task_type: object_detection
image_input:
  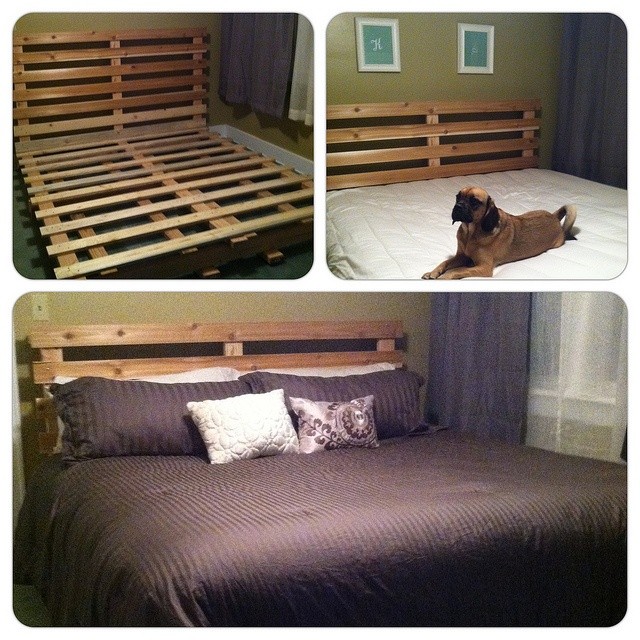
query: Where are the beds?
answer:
[328,101,628,280]
[14,31,315,282]
[30,325,628,627]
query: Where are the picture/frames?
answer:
[354,16,402,74]
[457,22,494,76]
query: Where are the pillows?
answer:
[187,387,300,464]
[51,376,254,462]
[288,395,379,453]
[239,367,424,438]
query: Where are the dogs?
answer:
[421,187,576,280]
